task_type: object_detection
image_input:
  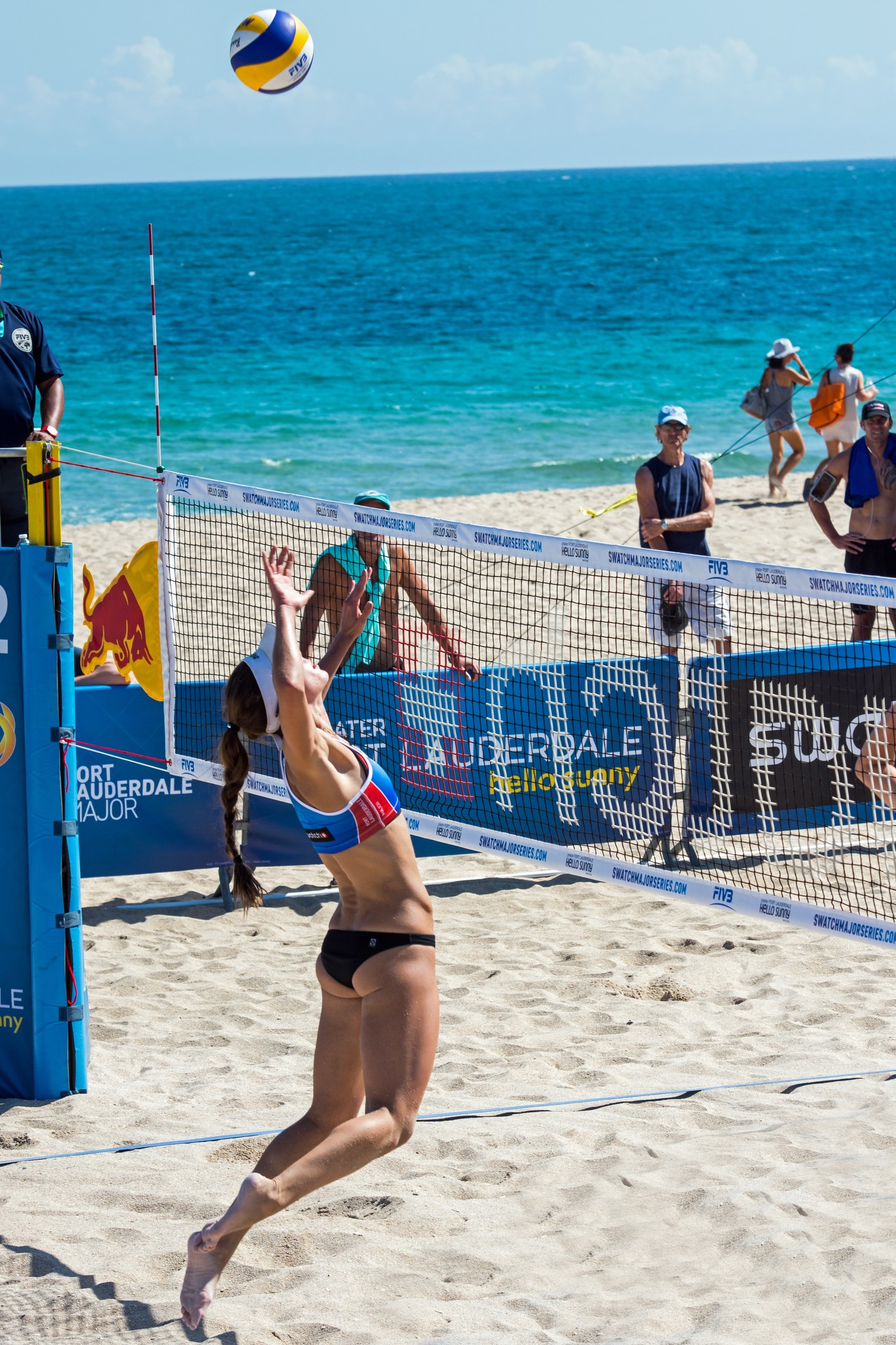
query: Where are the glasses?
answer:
[660,422,686,431]
[658,405,688,425]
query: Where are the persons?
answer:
[759,337,812,500]
[70,645,130,686]
[179,545,440,1330]
[0,248,66,549]
[802,343,878,503]
[300,490,483,683]
[807,401,896,643]
[636,405,732,656]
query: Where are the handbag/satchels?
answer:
[739,368,769,421]
[808,369,846,430]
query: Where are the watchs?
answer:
[661,518,668,532]
[40,427,58,440]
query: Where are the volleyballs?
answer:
[231,8,314,94]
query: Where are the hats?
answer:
[354,490,392,511]
[862,401,890,421]
[239,621,284,752]
[766,339,800,359]
